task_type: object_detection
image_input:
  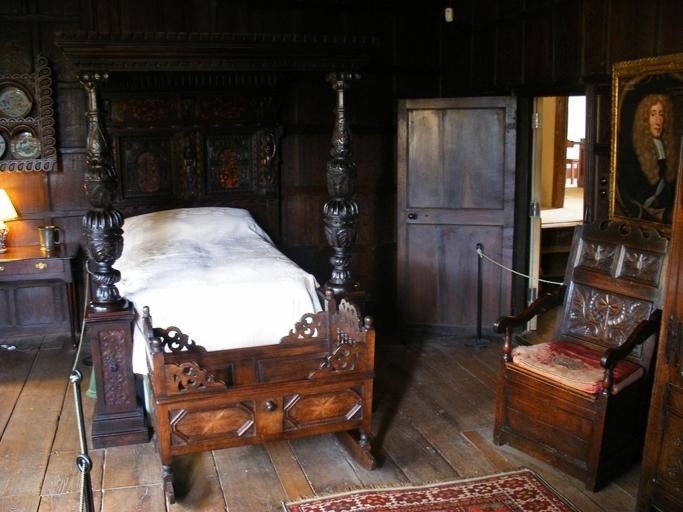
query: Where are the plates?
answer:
[0,125,41,161]
[0,82,34,121]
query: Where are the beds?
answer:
[113,208,378,504]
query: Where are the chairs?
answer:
[493,221,670,493]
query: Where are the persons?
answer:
[614,92,674,222]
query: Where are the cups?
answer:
[39,225,61,250]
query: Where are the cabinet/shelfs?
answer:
[526,225,575,332]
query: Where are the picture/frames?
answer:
[607,52,682,239]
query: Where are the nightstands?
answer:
[0,243,81,353]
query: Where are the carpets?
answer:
[279,465,584,512]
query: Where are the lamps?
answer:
[0,189,19,254]
